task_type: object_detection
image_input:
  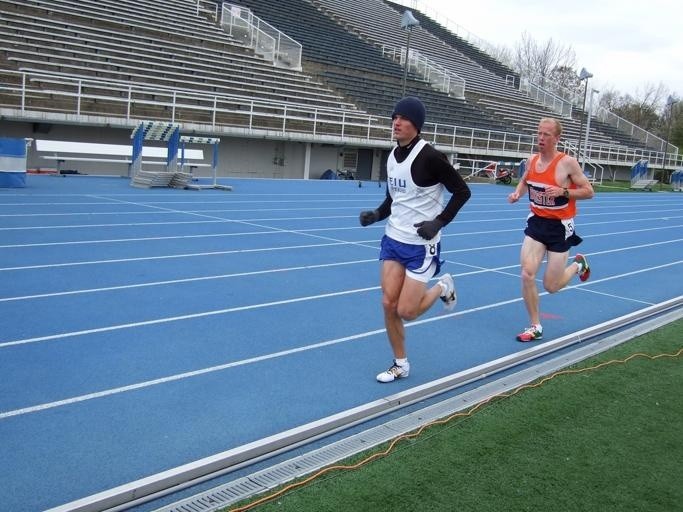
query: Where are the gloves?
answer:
[360,210,375,227]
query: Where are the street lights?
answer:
[578,65,593,156]
[662,94,677,185]
[400,9,419,99]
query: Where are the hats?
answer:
[391,96,427,135]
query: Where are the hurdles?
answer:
[131,119,233,191]
[495,158,530,178]
[629,159,657,192]
[670,169,683,192]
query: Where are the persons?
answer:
[508,117,596,342]
[360,97,473,384]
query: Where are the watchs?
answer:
[562,187,571,200]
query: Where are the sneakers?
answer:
[375,359,410,383]
[573,253,590,282]
[439,273,457,311]
[515,325,544,342]
[413,220,440,241]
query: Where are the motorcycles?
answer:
[485,163,512,184]
